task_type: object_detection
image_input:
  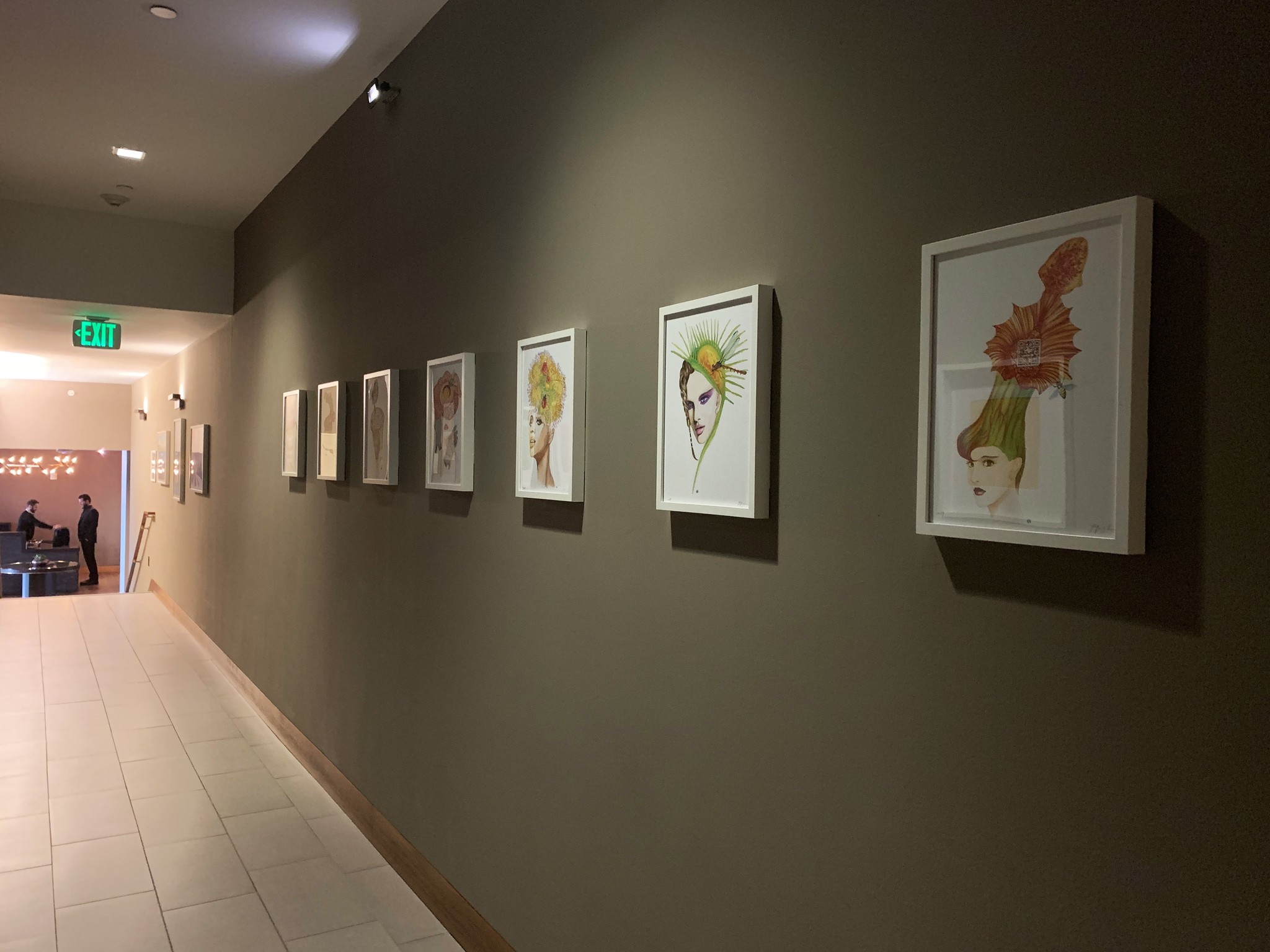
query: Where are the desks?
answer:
[0,558,81,597]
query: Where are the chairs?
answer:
[37,527,69,548]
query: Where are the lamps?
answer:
[0,452,80,478]
[168,394,184,409]
[135,409,146,420]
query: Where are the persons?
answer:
[16,499,62,542]
[78,494,99,586]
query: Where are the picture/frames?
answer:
[156,430,170,485]
[150,451,157,482]
[172,419,186,502]
[358,368,401,487]
[313,380,347,482]
[420,349,480,493]
[509,324,591,509]
[188,421,211,495]
[653,280,778,523]
[911,189,1163,562]
[279,389,307,479]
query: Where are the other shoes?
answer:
[80,578,96,585]
